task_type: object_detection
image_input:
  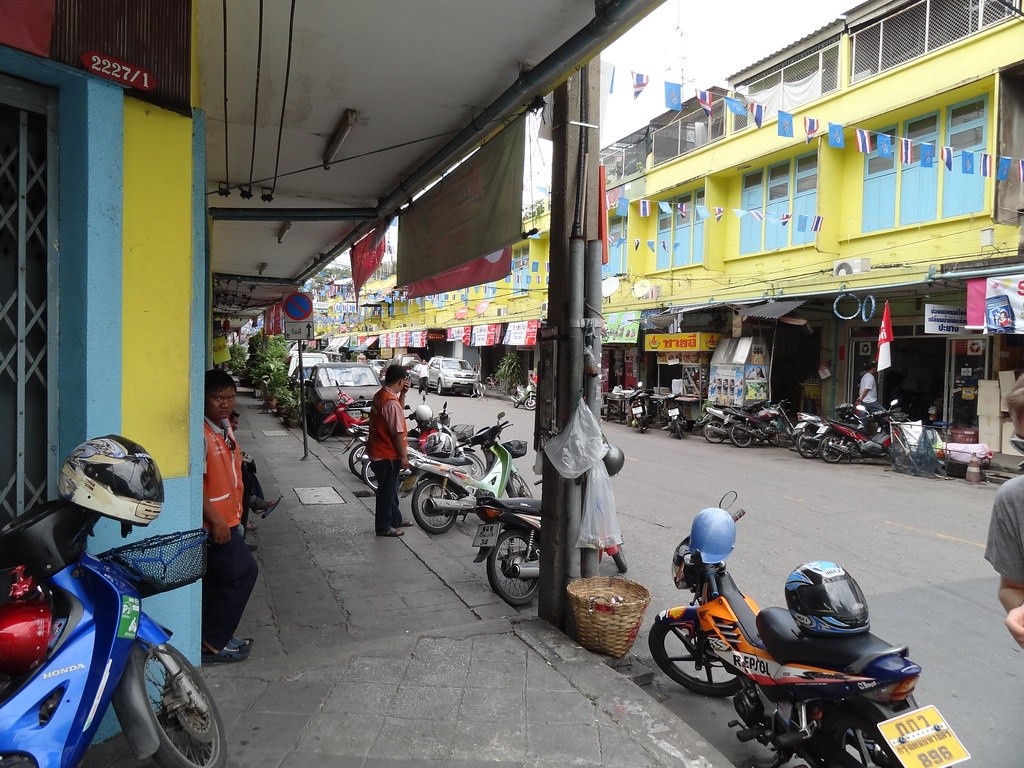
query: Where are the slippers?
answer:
[262,495,282,518]
[202,638,253,662]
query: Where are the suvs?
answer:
[305,361,383,436]
[427,355,480,397]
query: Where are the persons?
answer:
[709,367,766,399]
[984,374,1024,653]
[996,311,1011,326]
[202,369,259,664]
[417,359,431,395]
[856,364,888,435]
[366,365,414,537]
[228,411,282,551]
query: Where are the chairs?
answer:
[356,373,371,384]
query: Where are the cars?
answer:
[284,350,429,410]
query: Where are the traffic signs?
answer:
[284,321,314,341]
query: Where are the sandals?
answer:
[400,519,414,527]
[377,530,405,537]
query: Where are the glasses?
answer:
[1009,432,1024,454]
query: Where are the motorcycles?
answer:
[646,489,972,768]
[510,377,536,411]
[627,381,654,434]
[314,380,369,442]
[697,398,912,464]
[472,477,629,609]
[657,391,688,440]
[341,394,476,496]
[405,411,534,535]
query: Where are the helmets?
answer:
[59,435,164,526]
[689,508,736,563]
[0,600,51,672]
[786,562,869,636]
[427,432,452,457]
[416,405,432,420]
[603,444,624,475]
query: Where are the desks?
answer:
[602,390,698,425]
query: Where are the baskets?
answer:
[98,528,207,597]
[501,440,527,458]
[567,574,651,657]
[450,424,474,442]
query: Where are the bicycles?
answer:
[483,373,506,392]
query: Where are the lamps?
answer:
[259,262,268,275]
[323,108,357,171]
[277,222,291,244]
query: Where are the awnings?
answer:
[287,335,379,352]
[628,303,727,322]
[739,300,808,319]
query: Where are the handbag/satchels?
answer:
[576,461,623,548]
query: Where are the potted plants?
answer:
[495,352,524,396]
[227,337,306,427]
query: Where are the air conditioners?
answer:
[400,324,406,328]
[496,307,507,316]
[412,323,416,327]
[541,302,548,312]
[833,257,871,277]
[642,286,663,300]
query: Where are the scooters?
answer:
[1,499,232,768]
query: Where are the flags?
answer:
[876,302,894,372]
[599,60,1023,182]
[605,195,823,251]
[300,244,549,325]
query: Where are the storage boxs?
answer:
[977,369,1024,457]
